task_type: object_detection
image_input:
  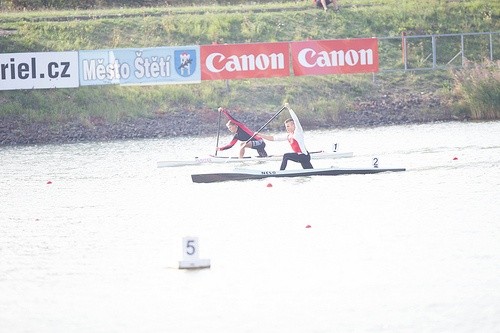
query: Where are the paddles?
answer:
[211,108,221,158]
[239,106,285,158]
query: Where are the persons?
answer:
[255,103,315,170]
[216,107,274,158]
[313,0,341,13]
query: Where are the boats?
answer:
[190,157,407,183]
[154,142,355,167]
